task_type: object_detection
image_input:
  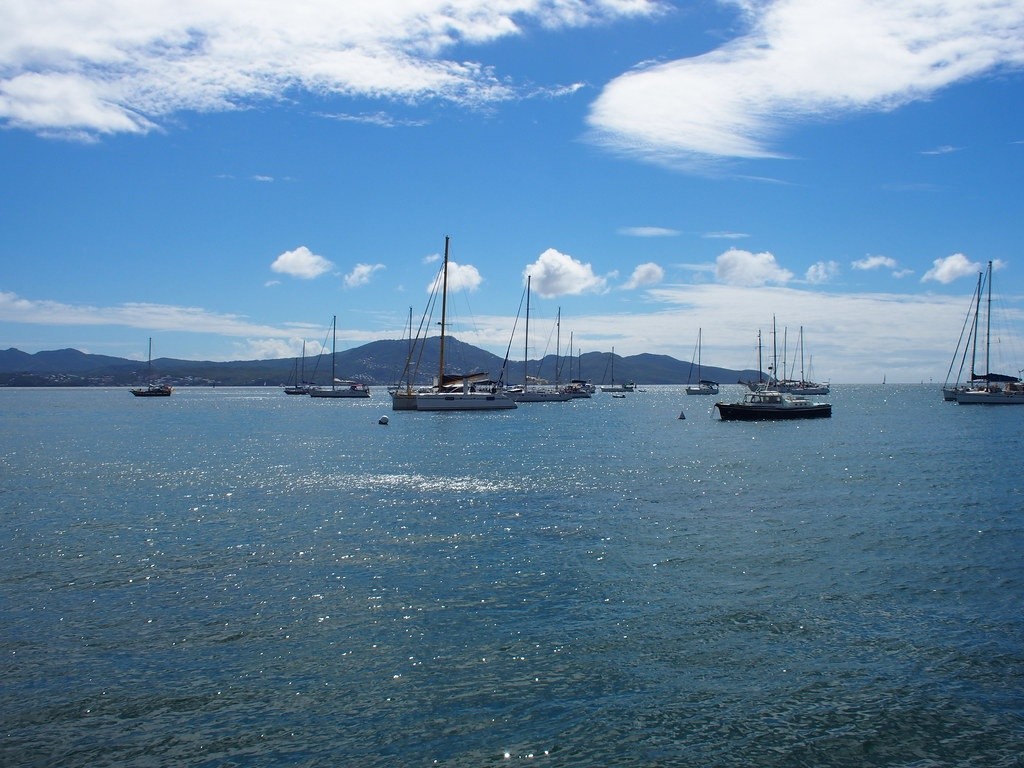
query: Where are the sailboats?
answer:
[388,235,454,410]
[128,337,172,396]
[307,316,373,398]
[599,346,634,392]
[684,328,719,396]
[492,275,572,402]
[285,358,303,389]
[956,261,1024,404]
[283,340,308,394]
[528,307,591,398]
[941,272,984,402]
[748,312,801,392]
[882,373,886,384]
[389,308,419,397]
[787,326,830,395]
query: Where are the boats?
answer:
[714,391,832,422]
[622,380,637,388]
[612,394,626,398]
[416,378,518,410]
[563,379,596,393]
[638,389,648,392]
